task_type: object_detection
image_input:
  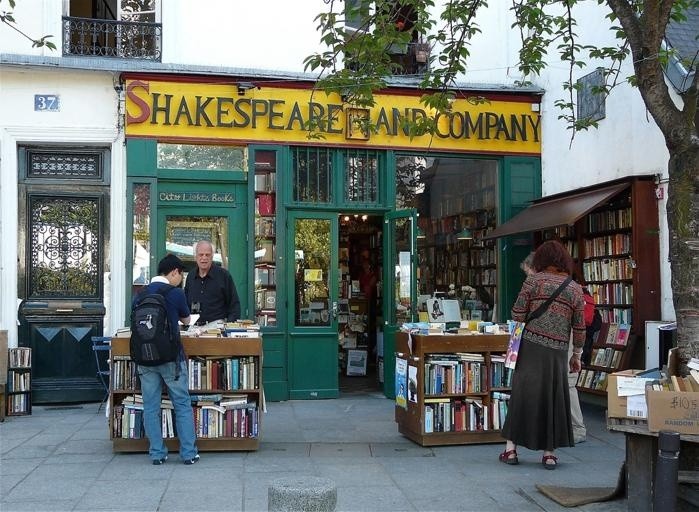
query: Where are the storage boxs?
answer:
[609,369,699,434]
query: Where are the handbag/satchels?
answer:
[505,321,525,370]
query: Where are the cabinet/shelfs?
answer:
[4,347,33,415]
[110,337,262,454]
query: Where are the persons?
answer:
[497,240,588,470]
[183,240,242,331]
[128,253,201,467]
[517,249,602,446]
[359,258,377,297]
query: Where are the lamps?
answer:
[237,80,254,93]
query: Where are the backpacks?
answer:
[129,284,180,366]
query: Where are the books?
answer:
[403,321,520,336]
[416,168,496,320]
[424,352,514,433]
[637,346,698,397]
[540,195,637,392]
[8,346,32,414]
[340,231,360,298]
[255,148,331,327]
[107,311,263,441]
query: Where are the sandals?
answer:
[541,455,557,469]
[499,449,518,464]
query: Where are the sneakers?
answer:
[151,456,168,465]
[184,454,200,464]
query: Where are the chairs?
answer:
[91,335,112,414]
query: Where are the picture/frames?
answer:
[165,222,217,261]
